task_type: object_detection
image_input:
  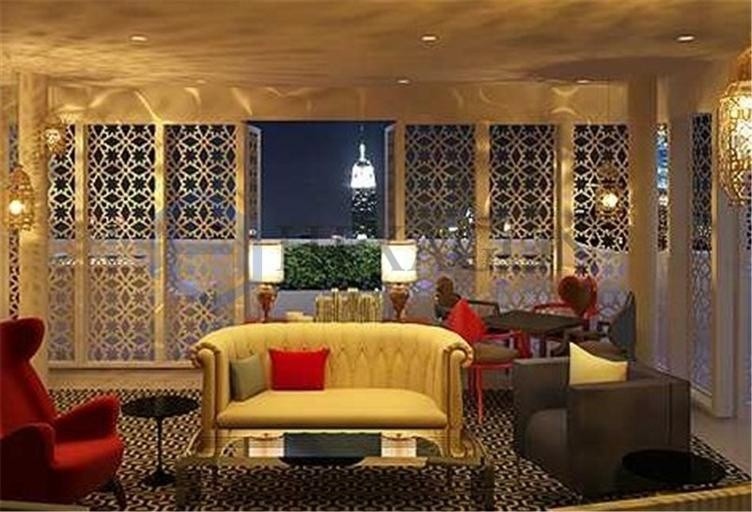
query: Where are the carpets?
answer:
[45,389,750,512]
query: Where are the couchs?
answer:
[191,322,474,459]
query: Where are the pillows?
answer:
[229,354,267,400]
[269,348,330,390]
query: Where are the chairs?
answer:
[435,275,636,423]
[513,354,689,497]
[0,319,124,511]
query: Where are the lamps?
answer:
[595,75,621,219]
[381,241,419,321]
[247,240,284,321]
[39,75,66,157]
[718,50,751,208]
[0,73,34,233]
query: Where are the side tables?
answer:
[122,396,199,487]
[619,449,726,493]
